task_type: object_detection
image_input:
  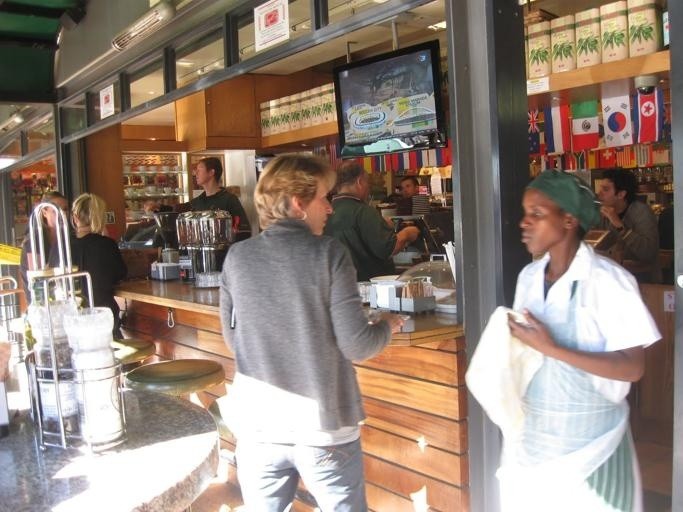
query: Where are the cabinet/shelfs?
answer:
[123,171,185,225]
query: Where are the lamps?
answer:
[14,113,24,124]
[111,0,177,53]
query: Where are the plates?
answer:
[369,274,413,282]
[411,192,431,217]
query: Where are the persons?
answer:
[19,191,71,313]
[592,167,661,284]
[55,193,124,342]
[217,153,405,511]
[323,158,419,281]
[399,177,419,214]
[497,170,647,512]
[143,154,250,268]
[657,202,675,251]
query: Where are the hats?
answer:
[526,170,600,232]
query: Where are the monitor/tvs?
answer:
[333,38,447,159]
[390,215,442,254]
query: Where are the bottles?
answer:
[0,288,122,446]
[661,1,670,51]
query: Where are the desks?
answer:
[0,388,220,512]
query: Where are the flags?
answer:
[526,87,662,155]
[313,144,451,170]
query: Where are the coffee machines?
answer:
[152,212,185,262]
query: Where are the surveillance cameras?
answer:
[634,74,660,95]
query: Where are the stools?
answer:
[124,359,226,400]
[110,338,156,366]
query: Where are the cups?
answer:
[123,163,181,218]
